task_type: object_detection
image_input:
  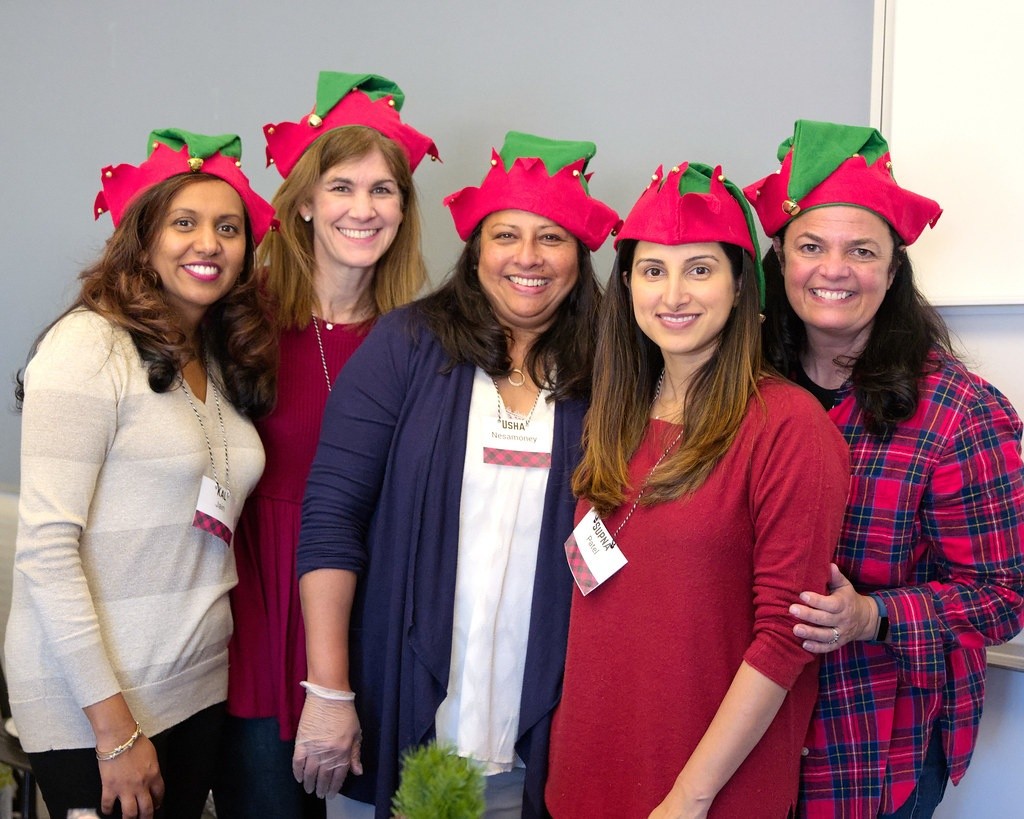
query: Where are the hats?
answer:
[613,161,766,323]
[741,118,943,247]
[263,71,443,183]
[94,128,282,252]
[442,129,619,252]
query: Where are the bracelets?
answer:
[95,720,142,761]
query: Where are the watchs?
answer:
[864,592,890,646]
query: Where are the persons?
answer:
[4,127,266,819]
[742,119,1024,819]
[210,71,444,819]
[293,130,625,819]
[544,161,851,819]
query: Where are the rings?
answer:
[828,628,839,645]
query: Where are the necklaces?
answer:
[312,295,380,330]
[507,369,526,387]
[655,370,686,419]
